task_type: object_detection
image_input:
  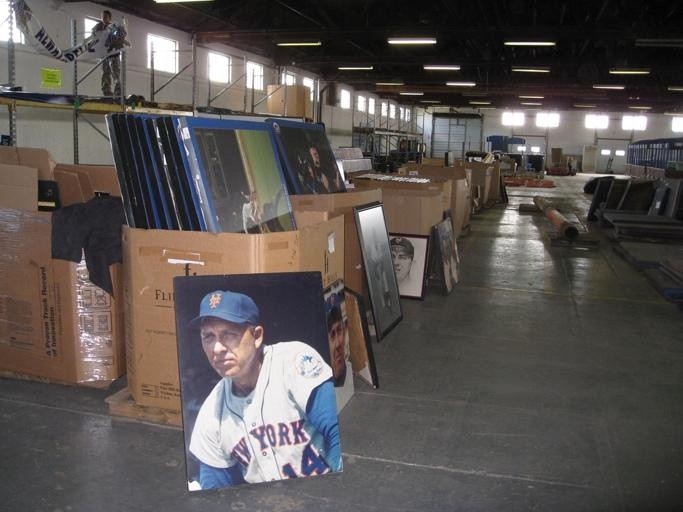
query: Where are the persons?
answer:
[325,306,355,415]
[187,289,343,490]
[389,237,418,295]
[309,145,328,176]
[440,235,458,294]
[91,10,121,96]
[329,286,339,306]
[370,225,394,314]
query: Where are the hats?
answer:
[191,290,262,328]
[390,236,415,253]
[325,294,342,314]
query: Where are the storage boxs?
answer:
[263,81,309,118]
